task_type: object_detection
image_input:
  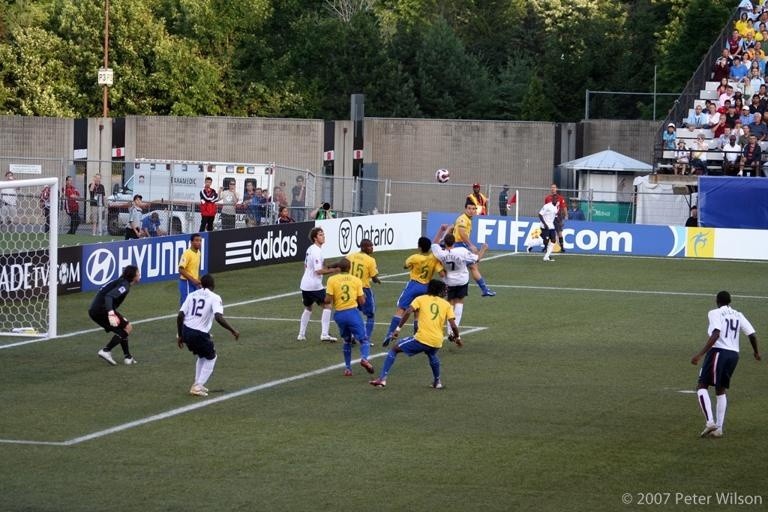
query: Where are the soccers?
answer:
[436,169,449,183]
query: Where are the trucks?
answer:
[97,157,275,237]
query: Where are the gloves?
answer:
[108,311,120,327]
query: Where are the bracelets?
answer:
[107,313,115,316]
[456,336,460,340]
[395,326,400,331]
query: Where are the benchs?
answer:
[658,48,767,176]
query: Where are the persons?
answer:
[367,277,464,390]
[88,264,143,365]
[346,238,383,347]
[39,184,61,232]
[525,194,561,263]
[542,184,569,253]
[323,258,375,376]
[88,173,107,237]
[124,193,144,240]
[383,235,447,348]
[140,212,162,238]
[685,205,698,228]
[175,275,240,397]
[430,223,488,347]
[179,234,201,309]
[296,227,337,342]
[0,171,22,226]
[662,0,768,177]
[567,198,585,221]
[691,291,761,438]
[61,176,82,235]
[464,181,488,216]
[497,184,510,215]
[198,172,337,240]
[450,201,497,297]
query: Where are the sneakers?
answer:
[430,384,442,389]
[98,349,116,366]
[344,369,352,376]
[481,291,496,296]
[527,245,532,253]
[383,335,392,347]
[321,335,337,343]
[192,385,208,397]
[448,334,455,342]
[543,257,555,262]
[361,360,374,373]
[297,335,306,341]
[124,355,137,365]
[369,380,386,387]
[711,431,723,438]
[701,425,718,438]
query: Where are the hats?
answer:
[677,140,686,145]
[473,184,480,187]
[742,106,749,110]
[668,123,675,128]
